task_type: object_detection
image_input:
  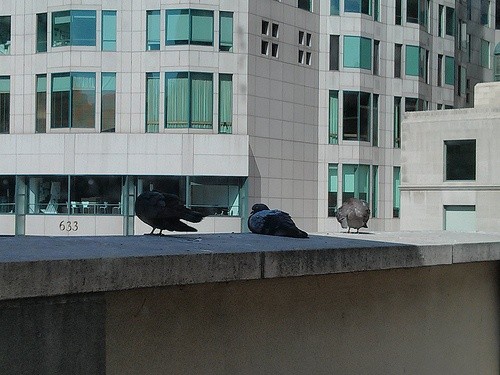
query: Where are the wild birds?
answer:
[134,190,208,237]
[247,204,308,238]
[335,197,370,235]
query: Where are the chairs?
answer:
[82,201,90,214]
[100,201,108,214]
[71,201,79,214]
[111,202,120,214]
